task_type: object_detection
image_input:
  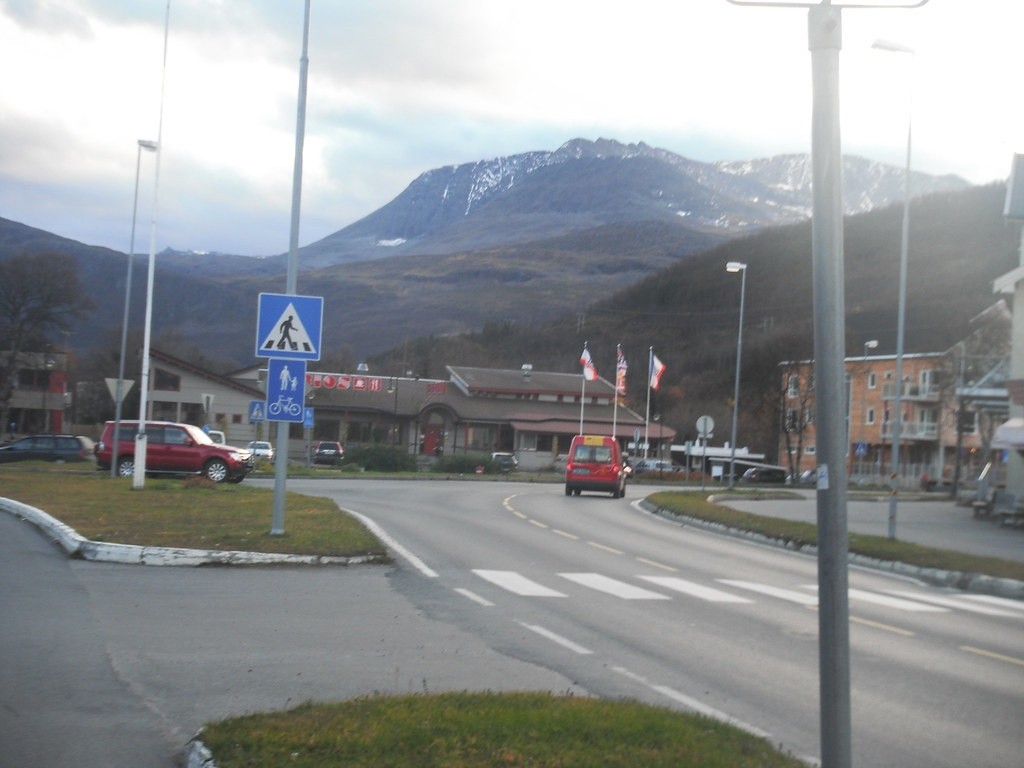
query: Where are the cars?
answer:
[0,435,95,461]
[627,457,687,473]
[246,441,276,463]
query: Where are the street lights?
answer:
[453,422,460,457]
[872,38,917,540]
[387,374,398,452]
[111,140,158,480]
[726,262,747,489]
[413,419,422,455]
[858,341,879,483]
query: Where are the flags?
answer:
[580,347,597,381]
[649,353,666,391]
[616,349,627,408]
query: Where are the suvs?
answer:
[490,452,518,472]
[314,441,344,464]
[97,421,254,483]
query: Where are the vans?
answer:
[565,435,627,498]
[205,431,225,445]
[742,468,786,484]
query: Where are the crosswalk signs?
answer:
[249,401,265,423]
[255,293,323,361]
